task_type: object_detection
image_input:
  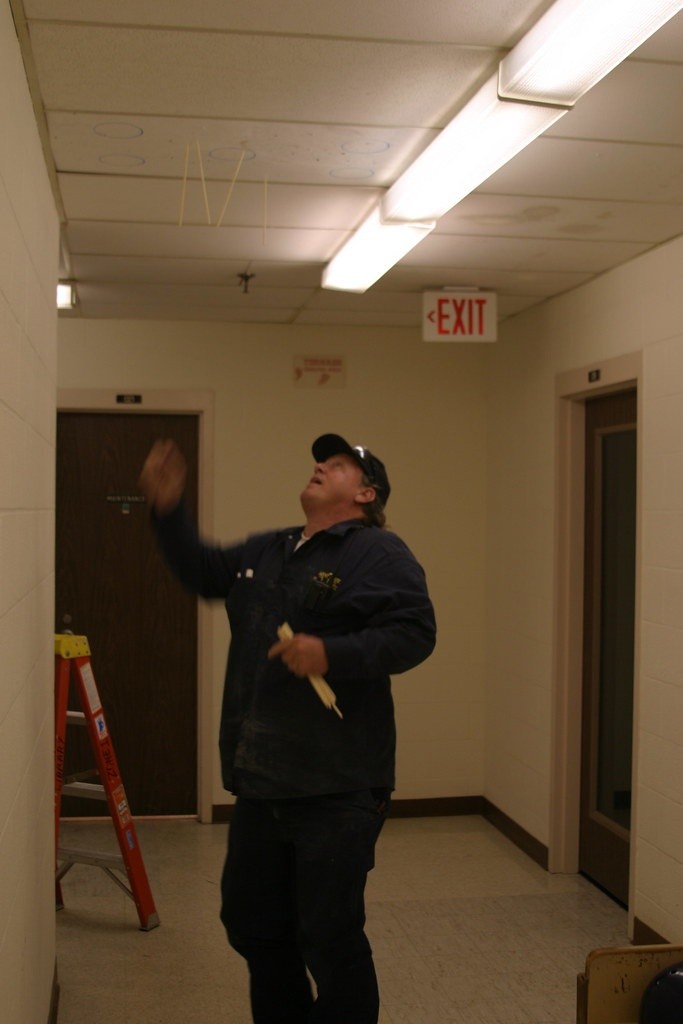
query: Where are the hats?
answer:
[312,433,391,504]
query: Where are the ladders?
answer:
[54,634,161,933]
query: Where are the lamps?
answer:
[317,0,682,294]
[56,279,78,310]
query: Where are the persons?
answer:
[141,433,438,1024]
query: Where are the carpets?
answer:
[362,890,634,1024]
